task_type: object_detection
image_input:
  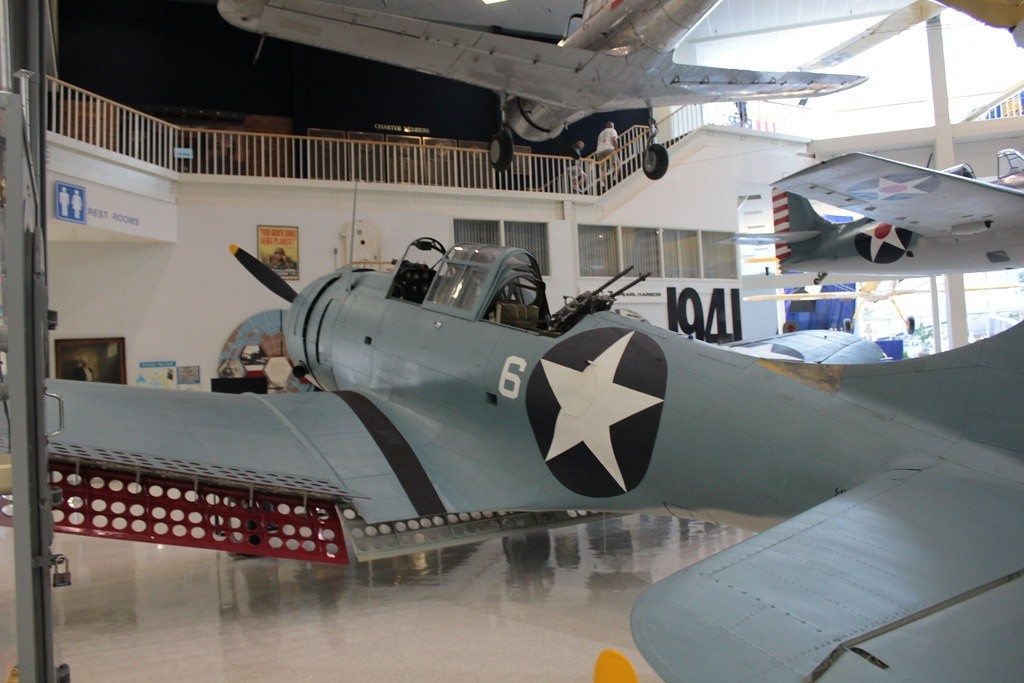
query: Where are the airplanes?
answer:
[720,146,1024,277]
[48,238,1024,683]
[216,0,871,180]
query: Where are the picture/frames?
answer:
[257,225,299,281]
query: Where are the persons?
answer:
[567,141,587,195]
[596,121,620,193]
